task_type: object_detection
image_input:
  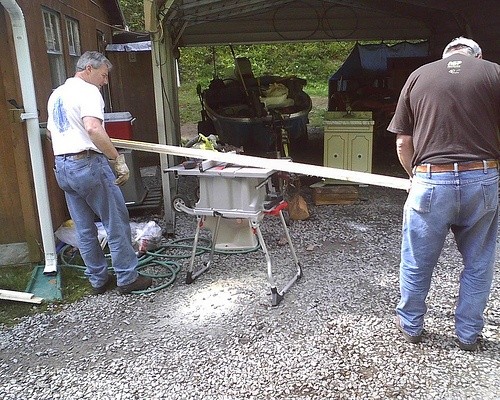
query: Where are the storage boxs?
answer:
[105,111,136,141]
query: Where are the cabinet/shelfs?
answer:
[320,109,375,187]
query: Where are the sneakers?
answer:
[121,273,151,293]
[92,274,115,294]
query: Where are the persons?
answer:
[387,35,499,351]
[47,50,153,296]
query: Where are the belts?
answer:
[59,150,99,156]
[415,160,497,173]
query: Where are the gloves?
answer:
[108,154,129,186]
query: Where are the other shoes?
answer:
[396,316,421,343]
[460,341,477,351]
